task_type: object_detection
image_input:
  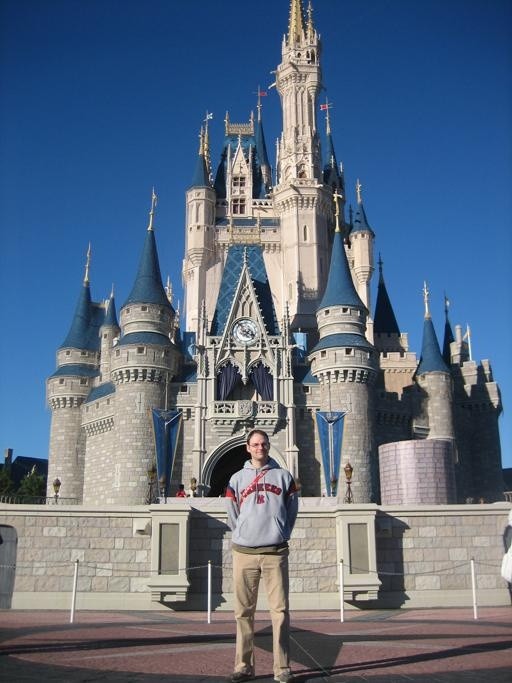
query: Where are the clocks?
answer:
[232,318,258,344]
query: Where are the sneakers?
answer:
[273,672,295,683]
[230,671,255,682]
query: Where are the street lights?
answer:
[148,465,155,504]
[344,462,355,506]
[53,478,63,502]
[159,472,170,504]
[191,477,197,495]
[329,474,337,497]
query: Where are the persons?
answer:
[225,431,298,683]
[185,478,198,497]
[176,484,186,498]
